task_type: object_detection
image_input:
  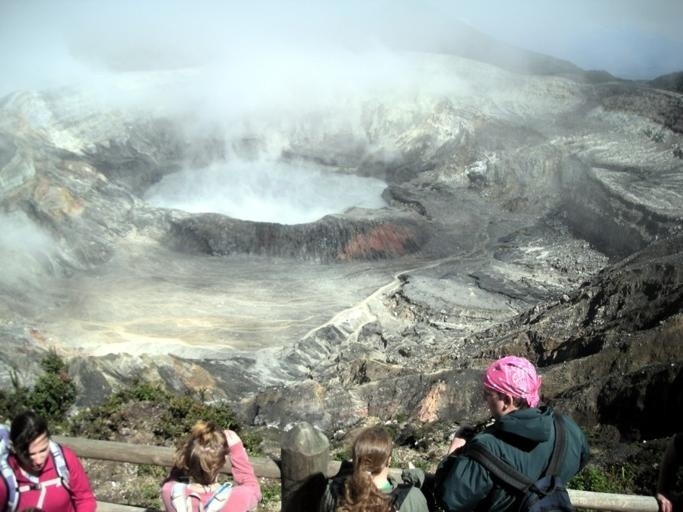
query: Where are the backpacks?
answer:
[459,411,573,511]
[1,426,70,512]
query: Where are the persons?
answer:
[431,355,590,512]
[0,411,98,512]
[318,426,431,512]
[160,419,262,512]
[654,430,683,512]
